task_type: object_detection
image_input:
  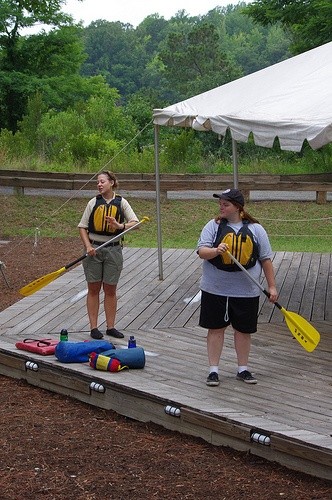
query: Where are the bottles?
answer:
[128,335,136,349]
[60,329,68,342]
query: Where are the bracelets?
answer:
[123,223,125,229]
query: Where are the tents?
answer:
[153,41,332,282]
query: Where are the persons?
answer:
[197,188,279,386]
[77,170,140,339]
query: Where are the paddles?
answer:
[224,248,321,352]
[19,216,150,297]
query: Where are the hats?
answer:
[213,189,245,206]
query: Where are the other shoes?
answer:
[91,328,103,339]
[206,372,220,385]
[236,370,258,384]
[106,328,124,338]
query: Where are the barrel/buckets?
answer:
[316,190,327,204]
